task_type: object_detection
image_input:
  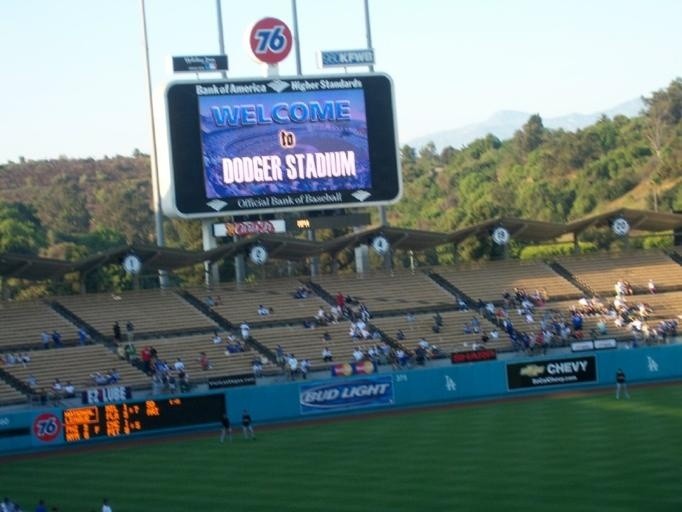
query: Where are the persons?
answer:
[349,317,409,364]
[416,338,444,364]
[36,499,48,512]
[91,368,118,386]
[99,496,112,512]
[504,286,608,351]
[456,296,512,350]
[303,292,370,329]
[298,285,308,298]
[607,291,675,346]
[240,408,257,441]
[6,351,38,384]
[649,279,657,294]
[42,327,86,348]
[276,345,311,379]
[0,495,15,512]
[208,295,223,307]
[119,344,190,394]
[616,368,630,400]
[212,321,269,377]
[432,311,442,334]
[323,347,332,362]
[258,305,268,315]
[12,504,23,512]
[324,331,331,341]
[200,352,209,371]
[52,378,75,400]
[112,321,135,342]
[407,312,416,320]
[616,279,634,294]
[218,412,233,444]
